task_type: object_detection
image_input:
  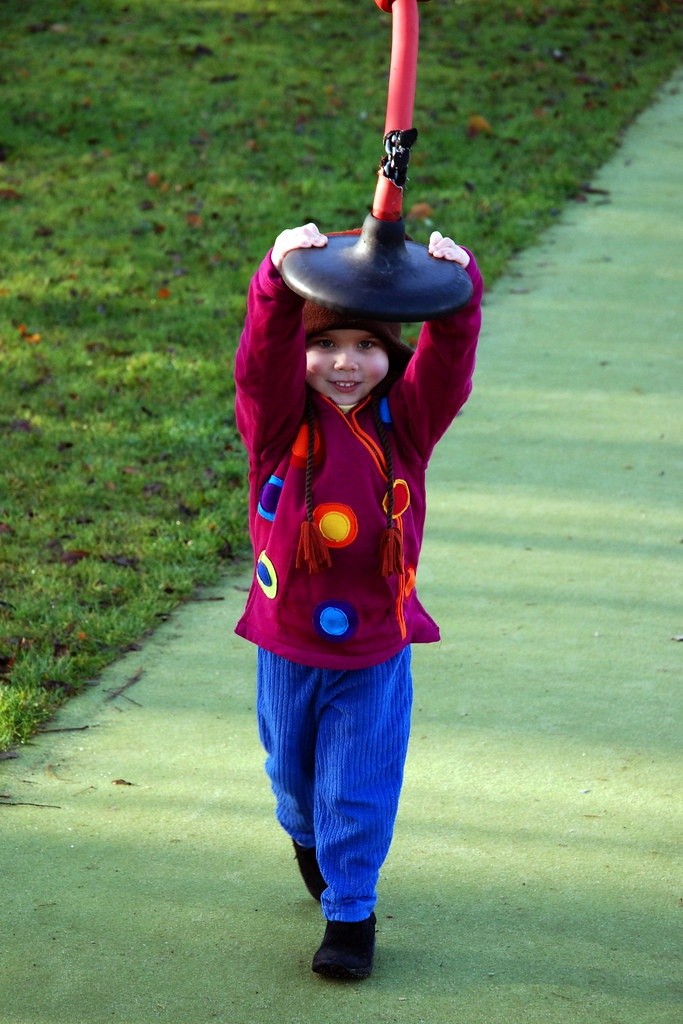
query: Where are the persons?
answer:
[234,220,483,981]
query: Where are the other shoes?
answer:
[292,839,328,904]
[311,910,377,979]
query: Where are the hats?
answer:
[301,298,418,578]
